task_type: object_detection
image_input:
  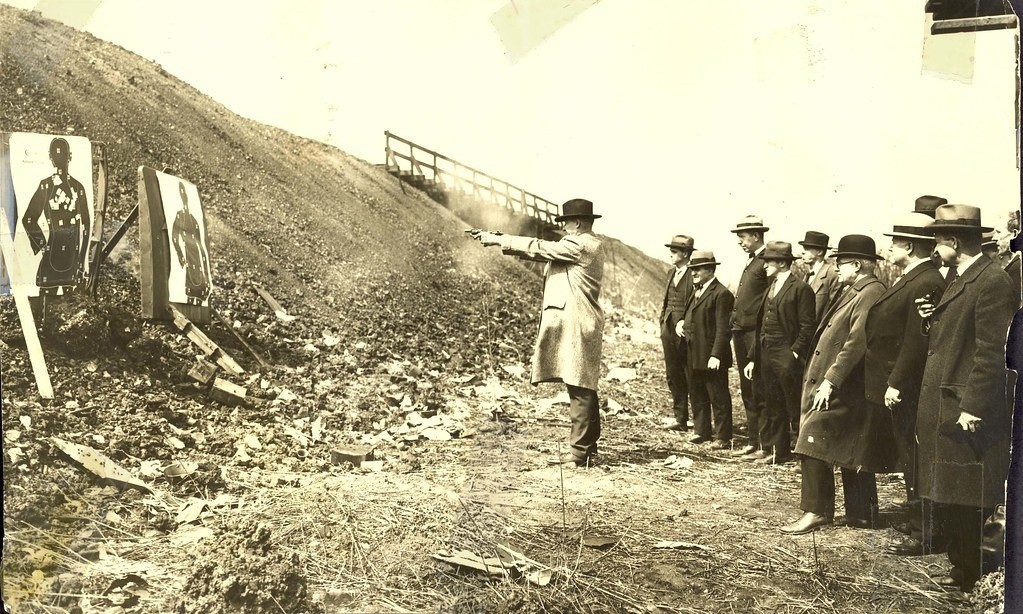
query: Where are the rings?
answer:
[890,401,894,405]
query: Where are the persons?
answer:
[660,235,734,449]
[475,199,604,465]
[731,195,1020,592]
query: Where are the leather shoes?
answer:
[931,576,971,602]
[892,497,922,510]
[888,520,911,536]
[663,420,688,431]
[688,433,705,443]
[779,511,828,534]
[709,438,734,449]
[731,445,789,464]
[832,514,872,528]
[886,536,944,556]
[546,451,592,466]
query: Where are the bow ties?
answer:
[695,283,703,290]
[749,253,755,258]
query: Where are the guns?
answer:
[465,228,484,239]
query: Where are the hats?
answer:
[981,232,996,246]
[664,235,698,251]
[910,196,948,213]
[731,214,770,233]
[924,204,994,232]
[686,250,721,268]
[798,231,832,249]
[828,234,885,261]
[758,241,802,260]
[555,198,602,222]
[883,212,936,240]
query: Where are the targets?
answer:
[8,131,95,297]
[154,168,215,308]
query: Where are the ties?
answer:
[770,278,778,299]
[891,274,905,287]
[805,271,814,283]
